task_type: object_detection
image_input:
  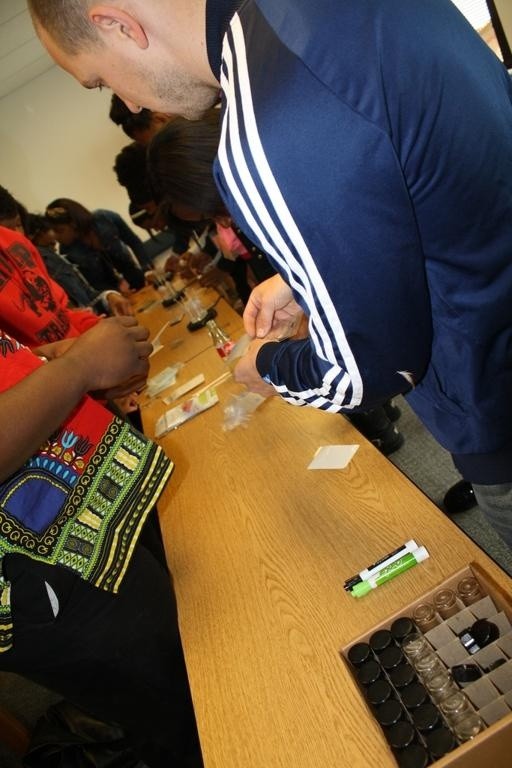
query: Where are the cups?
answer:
[174,284,209,324]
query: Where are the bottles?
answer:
[204,318,237,375]
[144,258,190,309]
[349,575,488,768]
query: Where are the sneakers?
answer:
[439,476,479,517]
[364,394,408,456]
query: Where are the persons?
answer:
[29,0,511,548]
[1,189,205,767]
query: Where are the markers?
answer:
[343,539,431,598]
